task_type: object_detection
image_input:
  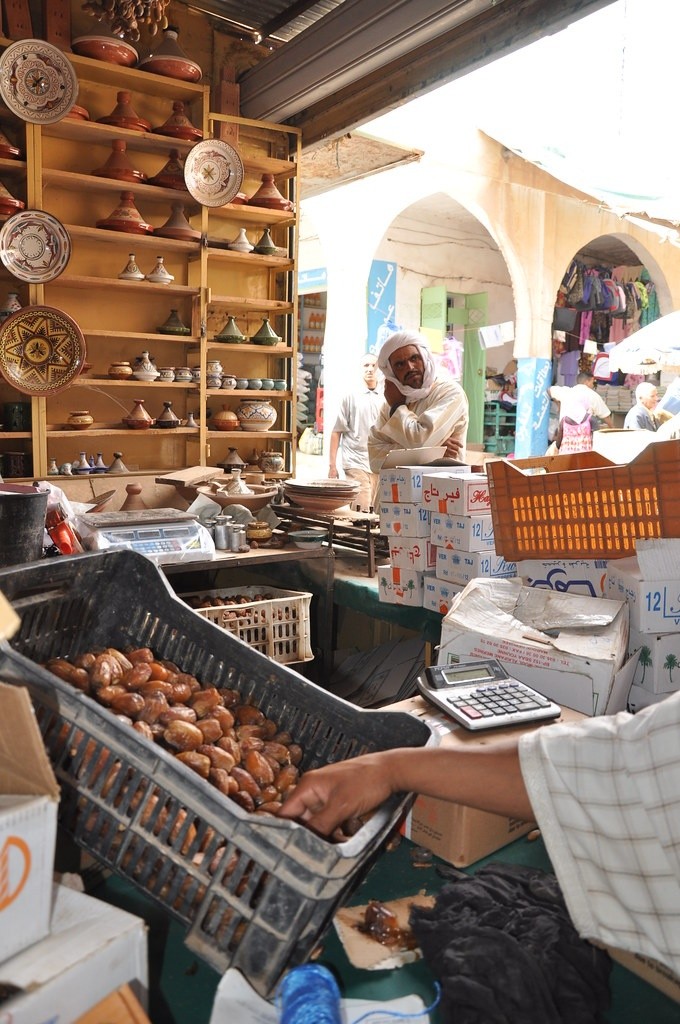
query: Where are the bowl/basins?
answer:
[139,55,203,84]
[286,530,328,548]
[71,35,139,68]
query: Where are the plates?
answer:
[281,477,362,514]
[0,38,78,124]
[183,138,244,207]
[0,208,72,284]
[0,305,87,396]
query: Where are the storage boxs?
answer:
[376,444,515,613]
[0,681,161,1024]
[0,550,439,1001]
[172,582,315,665]
[438,537,680,718]
[374,688,590,870]
[487,433,679,560]
[591,428,663,464]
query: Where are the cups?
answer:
[0,402,31,478]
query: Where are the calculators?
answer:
[417,658,563,733]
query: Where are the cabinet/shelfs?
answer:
[0,35,300,512]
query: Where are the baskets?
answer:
[176,583,315,668]
[484,440,680,562]
[0,547,435,1003]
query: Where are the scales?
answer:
[76,507,217,566]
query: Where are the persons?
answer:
[277,690,680,976]
[623,382,659,432]
[368,332,469,512]
[329,354,386,513]
[546,373,615,455]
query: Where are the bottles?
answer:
[48,458,58,475]
[246,521,272,546]
[204,514,246,553]
[106,351,287,390]
[0,292,24,326]
[256,452,285,474]
[66,410,94,429]
[275,963,343,1024]
